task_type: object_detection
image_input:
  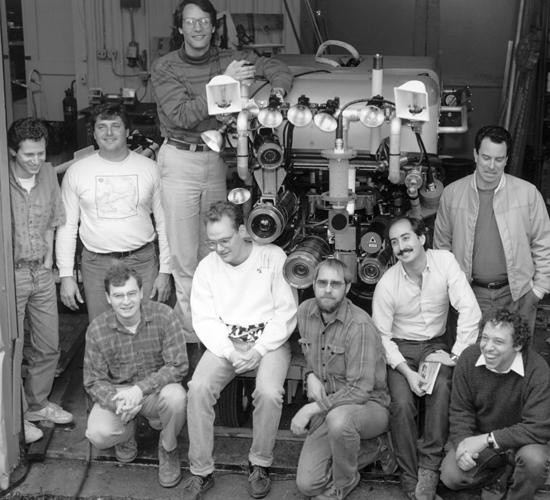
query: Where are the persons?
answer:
[55,103,173,324]
[290,260,391,500]
[441,308,550,500]
[7,117,73,443]
[432,124,550,349]
[180,201,298,500]
[152,0,293,360]
[83,267,190,489]
[371,216,483,500]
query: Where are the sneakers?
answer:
[312,471,361,500]
[481,464,514,500]
[379,432,398,475]
[159,433,181,487]
[115,417,137,462]
[26,401,73,423]
[407,482,443,500]
[24,419,44,444]
[416,467,439,499]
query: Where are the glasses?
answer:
[204,230,236,251]
[181,17,215,26]
[315,280,347,289]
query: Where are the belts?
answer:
[85,241,151,256]
[13,260,44,268]
[167,138,211,151]
[473,281,509,288]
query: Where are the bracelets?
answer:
[486,431,494,448]
[450,354,457,362]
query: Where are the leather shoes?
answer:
[182,472,215,499]
[247,459,272,499]
